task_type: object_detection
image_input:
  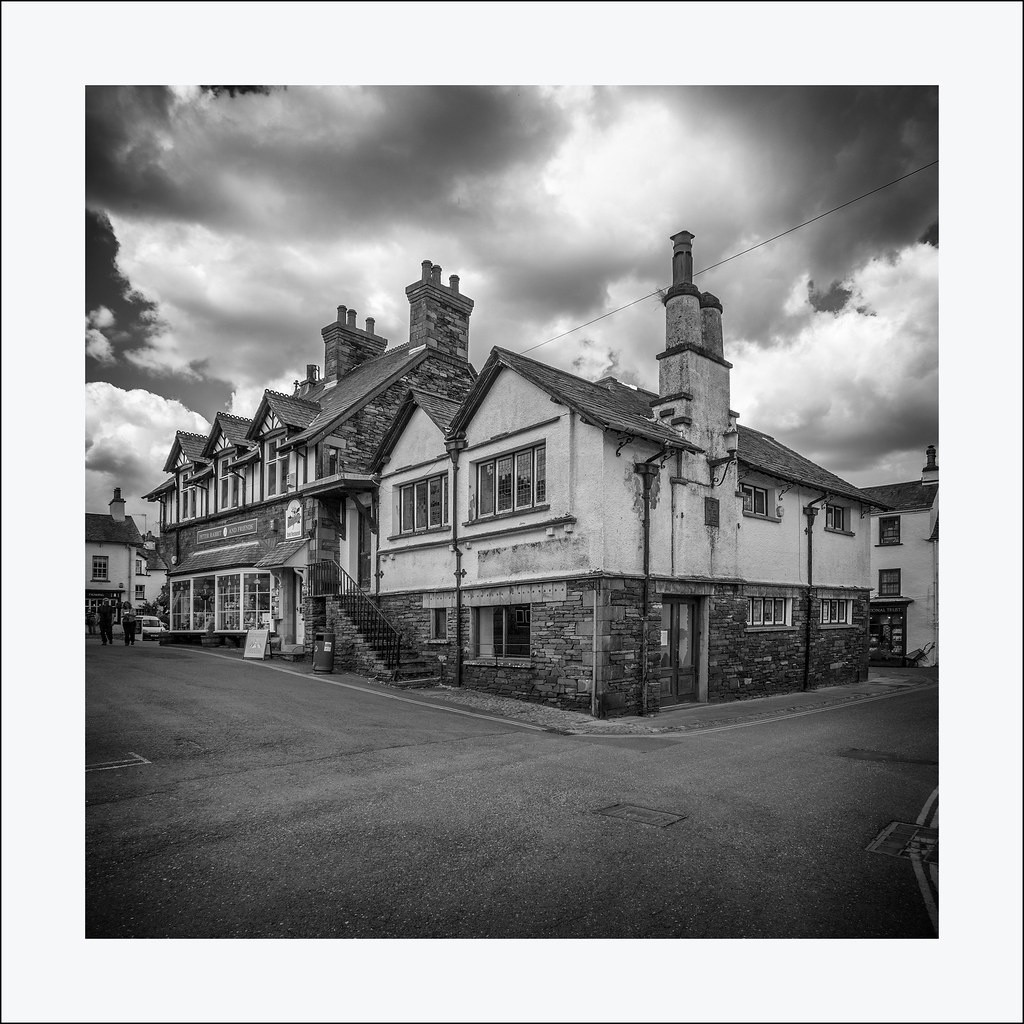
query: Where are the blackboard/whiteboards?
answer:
[243,630,272,659]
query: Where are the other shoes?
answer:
[93,633,97,634]
[125,643,129,646]
[109,639,112,644]
[131,642,134,645]
[102,642,106,645]
[90,632,93,635]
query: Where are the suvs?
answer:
[135,615,166,639]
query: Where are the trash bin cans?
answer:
[312,633,336,675]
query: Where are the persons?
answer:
[120,601,136,645]
[98,598,115,646]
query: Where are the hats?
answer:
[103,598,109,601]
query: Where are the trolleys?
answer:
[904,642,935,667]
